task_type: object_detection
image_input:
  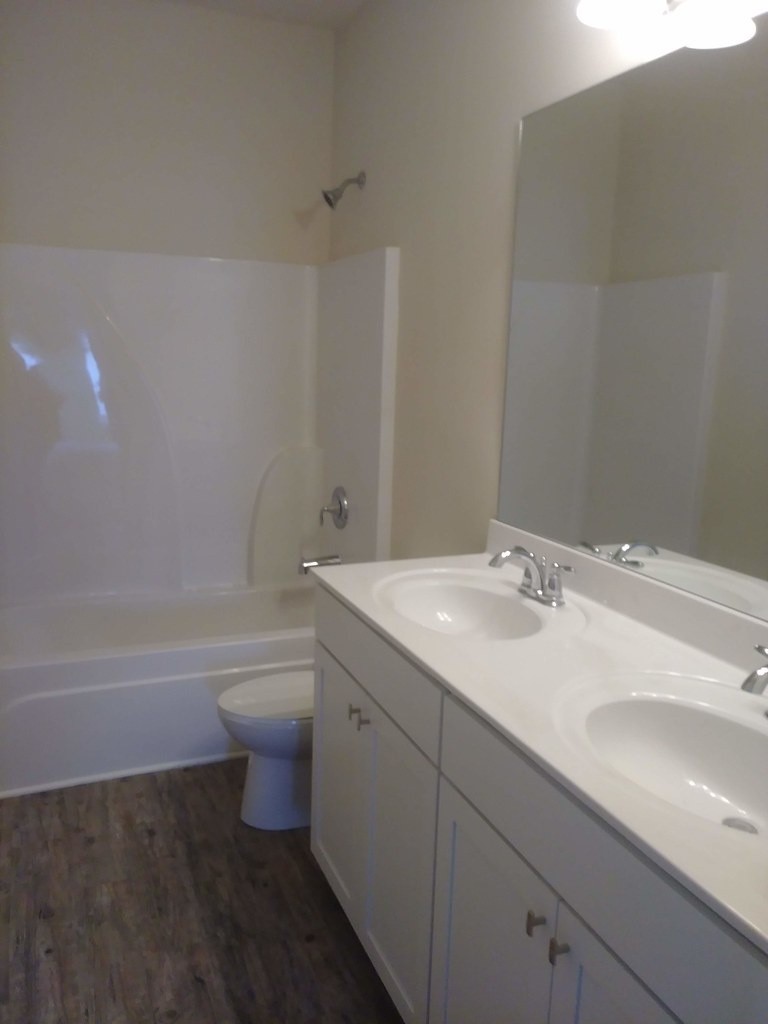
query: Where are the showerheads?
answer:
[322,186,347,212]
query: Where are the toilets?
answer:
[216,667,318,833]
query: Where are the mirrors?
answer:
[491,9,766,622]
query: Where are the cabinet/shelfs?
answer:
[433,679,764,1023]
[299,569,443,1022]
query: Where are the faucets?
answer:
[297,555,347,575]
[611,539,664,574]
[487,546,550,603]
[737,644,768,695]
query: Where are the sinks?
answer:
[583,691,768,846]
[390,581,543,650]
[620,571,757,620]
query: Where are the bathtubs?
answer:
[0,625,318,802]
